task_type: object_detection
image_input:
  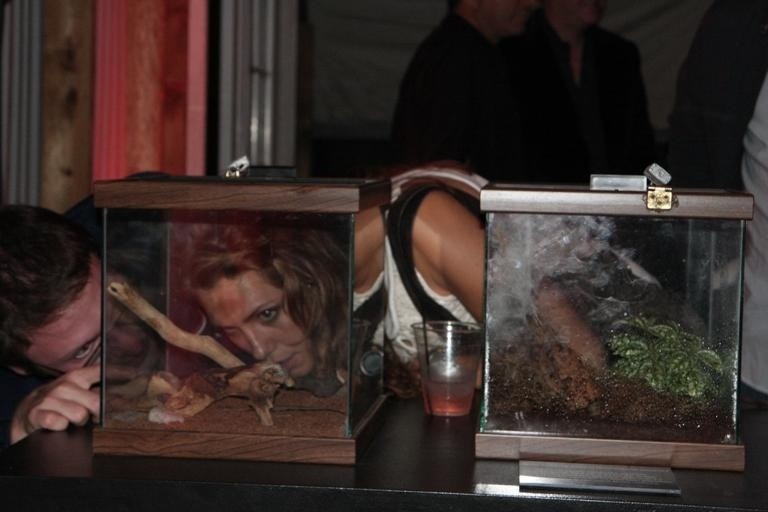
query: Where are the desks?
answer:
[0,379,767,512]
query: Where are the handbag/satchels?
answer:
[461,250,715,352]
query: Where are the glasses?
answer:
[28,306,128,374]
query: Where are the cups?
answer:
[410,320,483,417]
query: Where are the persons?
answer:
[0,174,194,452]
[730,69,767,405]
[502,0,655,182]
[192,168,705,390]
[392,1,546,181]
